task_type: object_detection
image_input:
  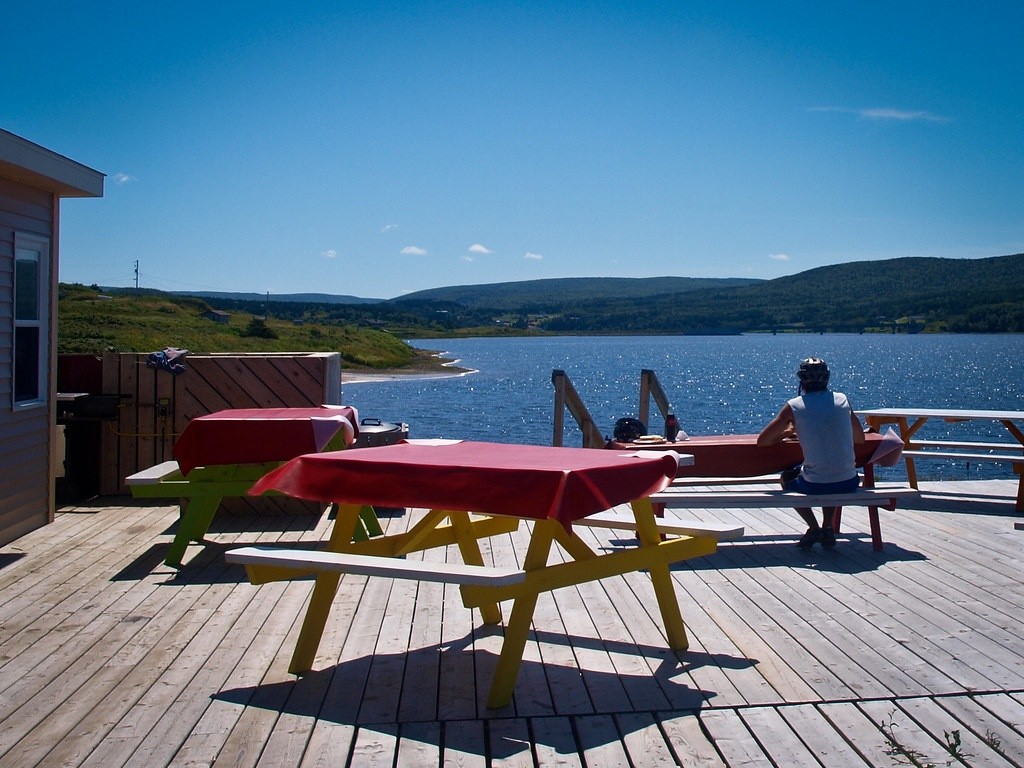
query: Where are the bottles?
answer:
[667,403,677,442]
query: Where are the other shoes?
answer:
[798,528,836,549]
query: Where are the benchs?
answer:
[670,472,866,485]
[648,487,919,507]
[225,545,528,590]
[470,507,746,540]
[900,450,1024,464]
[124,460,181,483]
[908,439,1024,451]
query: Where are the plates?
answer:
[633,439,667,444]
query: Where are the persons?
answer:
[757,357,865,548]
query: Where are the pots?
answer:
[359,418,402,435]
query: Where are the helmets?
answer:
[797,358,830,383]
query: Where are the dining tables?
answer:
[244,437,718,710]
[130,406,384,566]
[853,407,1024,513]
[613,431,903,551]
